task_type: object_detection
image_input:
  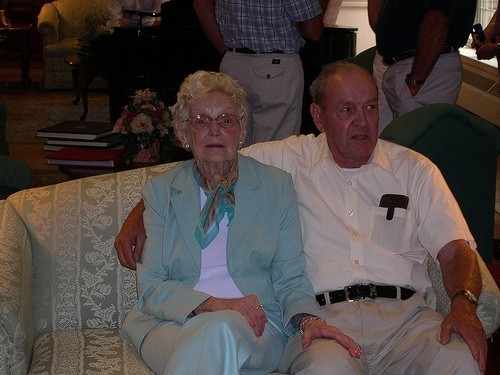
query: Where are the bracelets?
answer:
[405,78,426,85]
[299,315,320,335]
[496,43,500,51]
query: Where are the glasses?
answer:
[181,114,243,129]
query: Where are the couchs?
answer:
[0,160,500,375]
[38,0,121,94]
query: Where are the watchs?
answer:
[450,289,478,308]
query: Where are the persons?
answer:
[121,69,363,375]
[114,62,488,375]
[193,0,324,150]
[470,0,500,87]
[364,0,479,137]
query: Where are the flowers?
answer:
[111,89,178,164]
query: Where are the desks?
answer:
[58,143,190,181]
[64,55,112,120]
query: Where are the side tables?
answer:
[0,24,35,92]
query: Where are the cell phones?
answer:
[471,23,486,43]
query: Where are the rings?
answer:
[255,304,264,312]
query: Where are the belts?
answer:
[384,47,457,66]
[226,47,284,55]
[315,283,415,306]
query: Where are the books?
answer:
[36,120,126,170]
